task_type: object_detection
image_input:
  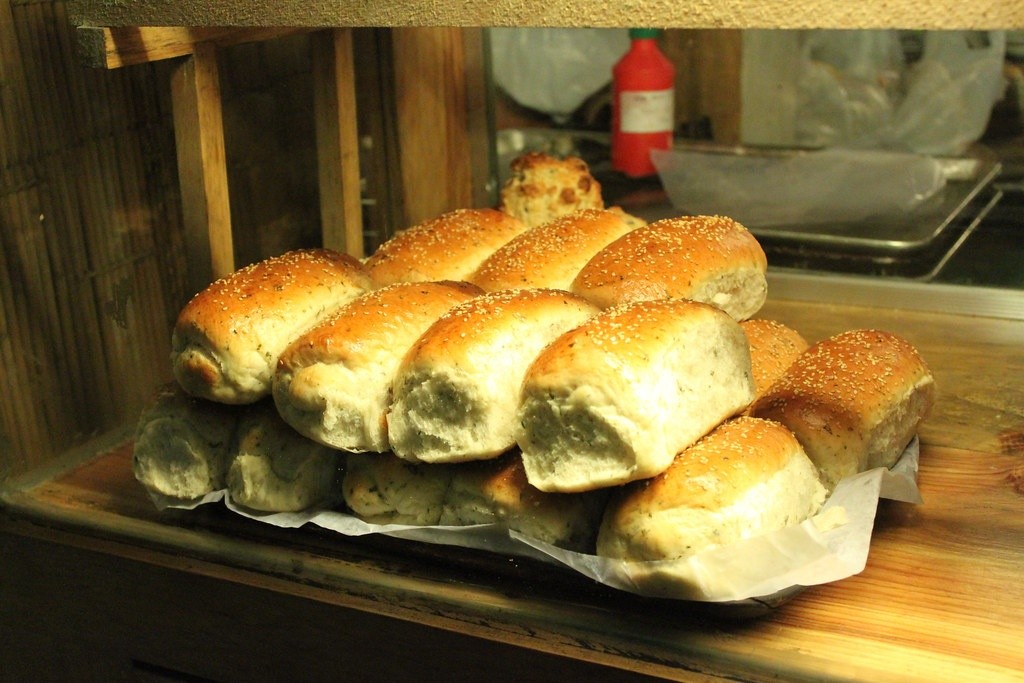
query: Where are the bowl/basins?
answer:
[649,143,847,230]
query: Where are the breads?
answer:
[134,149,934,600]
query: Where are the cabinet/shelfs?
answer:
[0,0,1024,683]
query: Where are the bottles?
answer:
[613,28,676,177]
